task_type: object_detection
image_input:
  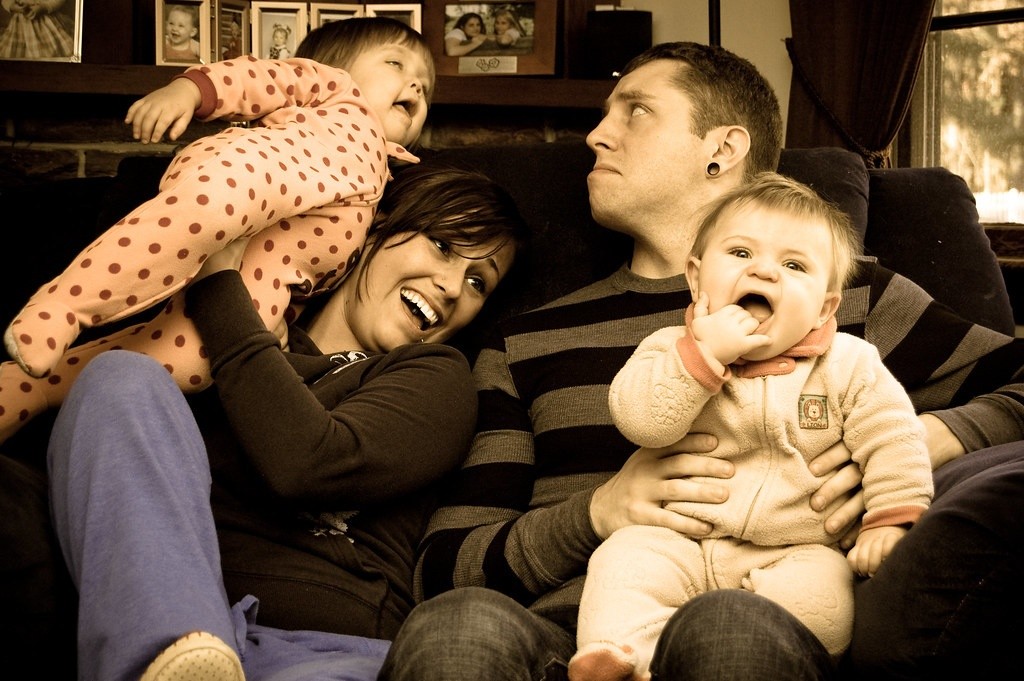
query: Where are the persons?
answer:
[444,10,526,55]
[0,16,437,447]
[377,42,1024,681]
[49,163,525,681]
[0,0,292,62]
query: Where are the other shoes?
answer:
[139,632,246,681]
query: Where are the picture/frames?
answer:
[0,0,85,63]
[366,4,422,36]
[252,2,307,62]
[311,3,364,31]
[422,0,558,77]
[155,0,211,66]
[211,0,251,63]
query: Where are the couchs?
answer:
[0,146,1024,681]
